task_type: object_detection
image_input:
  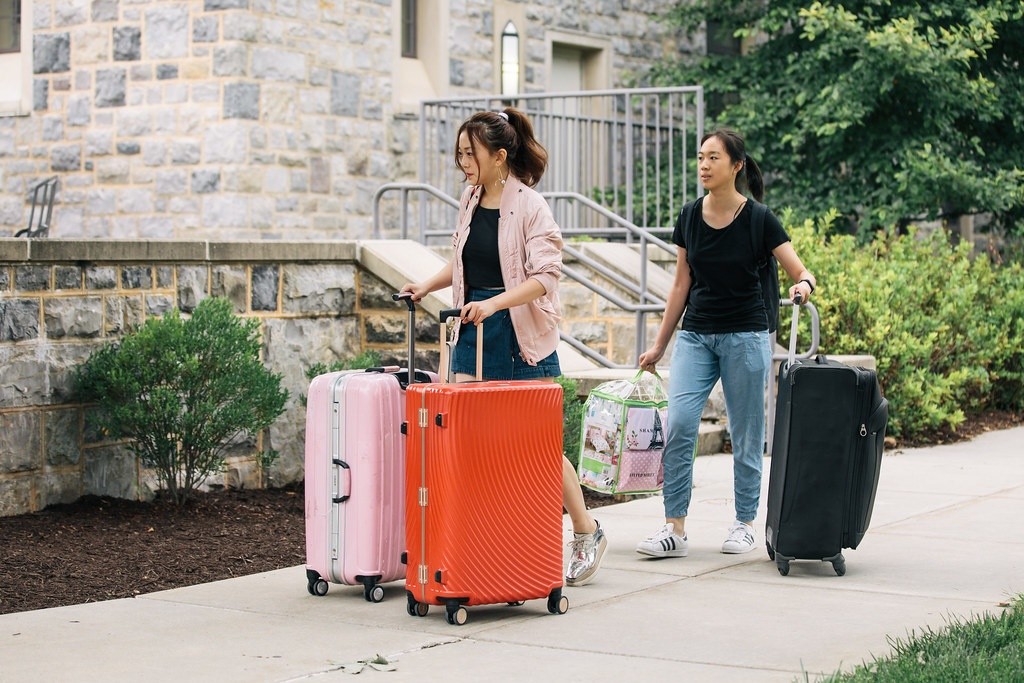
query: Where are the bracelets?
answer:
[798,278,815,295]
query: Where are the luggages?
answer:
[400,309,569,625]
[765,293,889,577]
[304,292,438,603]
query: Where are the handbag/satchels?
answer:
[576,368,698,495]
[438,342,456,383]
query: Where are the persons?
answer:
[401,107,606,588]
[636,131,816,557]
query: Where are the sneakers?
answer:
[565,518,608,586]
[636,522,688,556]
[720,520,758,553]
